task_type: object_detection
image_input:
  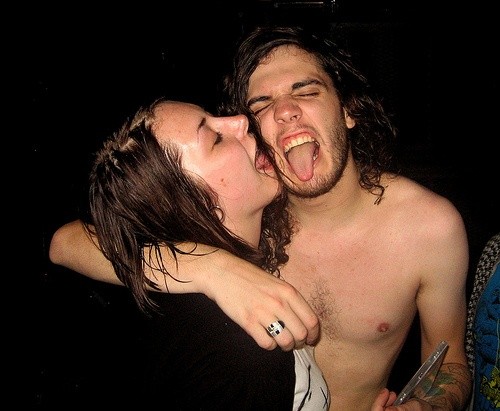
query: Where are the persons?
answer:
[82,95,332,411]
[46,23,471,411]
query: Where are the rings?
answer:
[264,320,284,336]
